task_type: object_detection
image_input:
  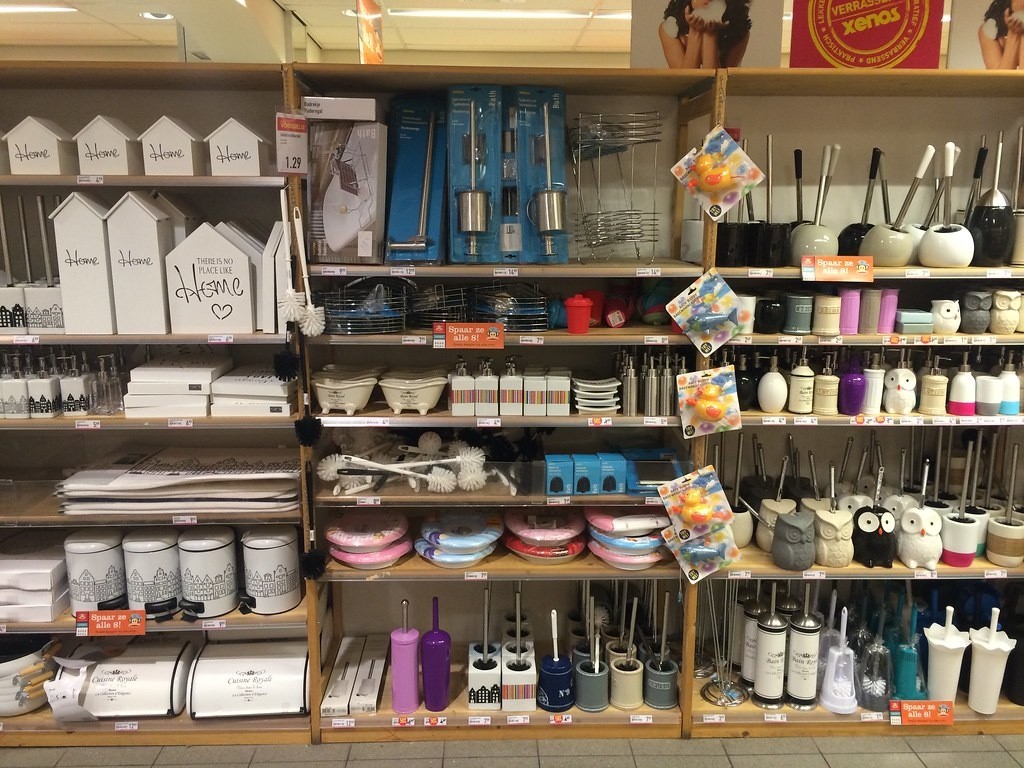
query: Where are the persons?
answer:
[658,0,753,69]
[977,0,1024,70]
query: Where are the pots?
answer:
[0,637,61,716]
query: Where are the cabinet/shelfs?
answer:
[0,62,1024,748]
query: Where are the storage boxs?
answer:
[320,636,366,717]
[895,322,934,334]
[571,454,601,495]
[896,308,934,324]
[349,634,391,714]
[597,453,627,494]
[544,454,574,497]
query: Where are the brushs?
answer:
[902,426,1024,533]
[317,428,555,495]
[817,579,926,699]
[276,187,326,338]
[470,578,674,677]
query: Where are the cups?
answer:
[878,287,898,334]
[859,289,882,334]
[783,292,813,335]
[812,295,842,336]
[566,296,593,333]
[838,289,862,334]
[738,295,756,334]
